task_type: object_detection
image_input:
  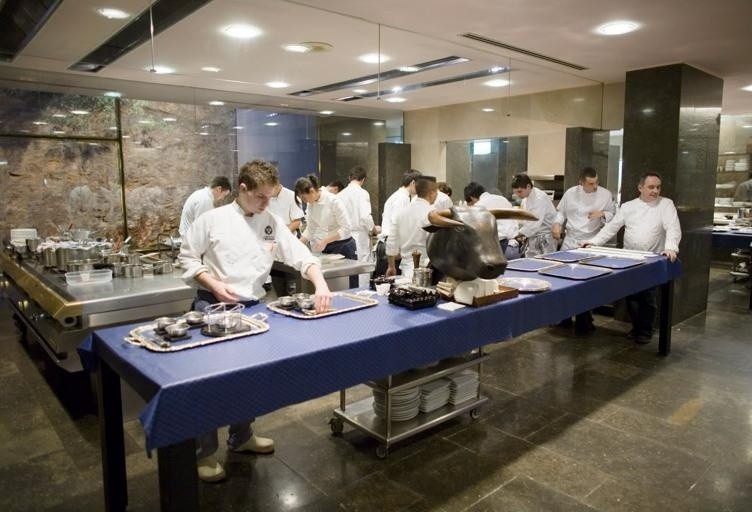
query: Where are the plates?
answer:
[372,388,421,422]
[419,378,450,413]
[267,290,380,320]
[731,227,752,234]
[498,277,551,292]
[734,162,747,171]
[534,249,606,263]
[124,312,270,353]
[580,255,648,270]
[447,370,480,406]
[505,257,563,272]
[538,263,612,280]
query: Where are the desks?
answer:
[76,246,683,512]
[269,251,375,299]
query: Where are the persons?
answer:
[178,176,232,239]
[374,168,423,277]
[577,173,683,360]
[463,181,521,260]
[337,166,379,289]
[266,179,303,239]
[511,175,559,259]
[384,175,438,276]
[319,180,345,194]
[294,174,360,290]
[433,181,454,210]
[178,160,333,482]
[550,166,614,338]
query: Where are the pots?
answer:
[107,251,161,265]
[55,246,100,272]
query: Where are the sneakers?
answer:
[636,330,653,344]
[626,329,636,339]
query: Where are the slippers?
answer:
[196,456,226,482]
[227,434,274,453]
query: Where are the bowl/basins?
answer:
[67,260,87,272]
[122,264,143,278]
[724,159,735,171]
[153,261,173,274]
[296,300,314,309]
[292,292,310,300]
[279,297,297,309]
[155,317,175,331]
[166,323,190,337]
[104,262,126,277]
[184,312,205,326]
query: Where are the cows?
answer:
[422,207,539,285]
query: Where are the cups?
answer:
[414,268,433,287]
